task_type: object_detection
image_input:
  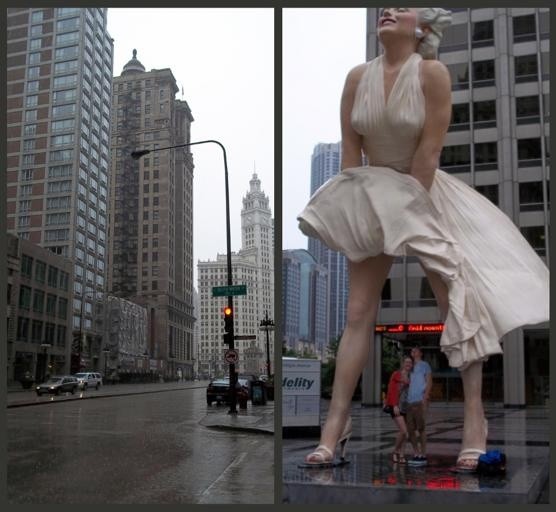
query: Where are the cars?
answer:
[206,376,241,406]
[224,370,267,398]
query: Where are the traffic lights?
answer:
[223,334,232,344]
[222,308,232,334]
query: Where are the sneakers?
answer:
[408,456,414,464]
[413,456,428,464]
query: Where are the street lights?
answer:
[128,139,239,413]
[260,310,273,379]
[100,346,109,386]
[39,340,54,383]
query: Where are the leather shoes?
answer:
[400,455,407,463]
[392,451,398,463]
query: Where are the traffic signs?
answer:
[211,283,247,299]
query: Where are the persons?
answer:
[404,346,433,461]
[299,8,552,471]
[383,359,413,463]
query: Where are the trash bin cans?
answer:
[249,380,267,406]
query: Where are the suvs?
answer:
[35,376,79,396]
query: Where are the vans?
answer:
[72,372,104,390]
